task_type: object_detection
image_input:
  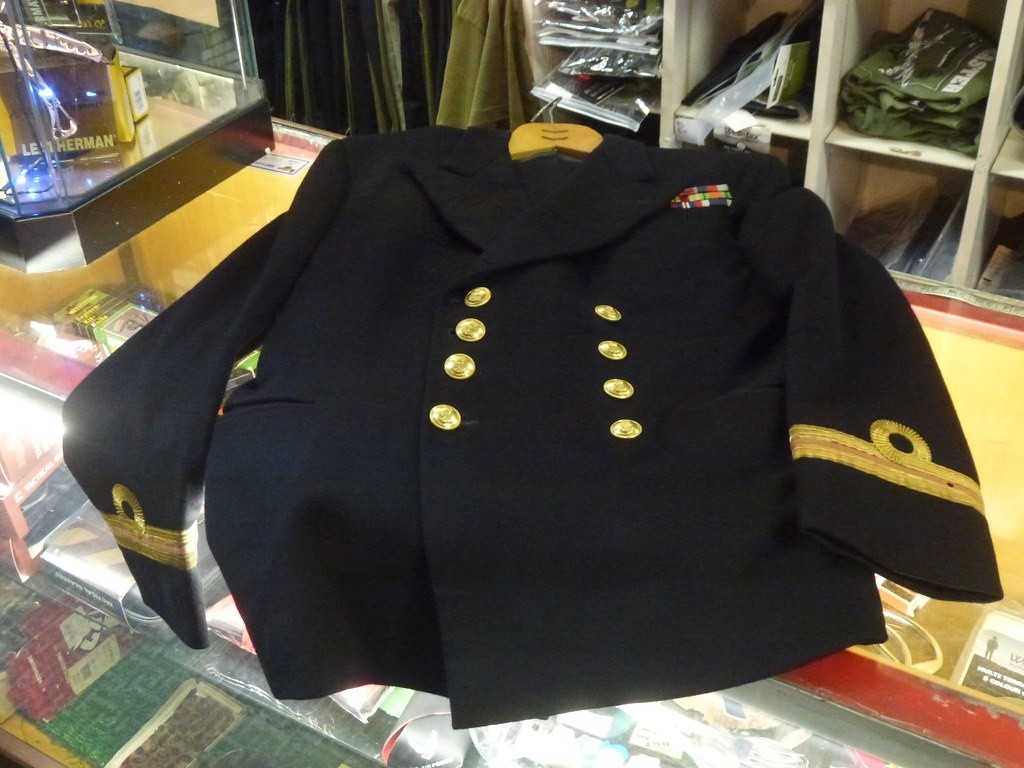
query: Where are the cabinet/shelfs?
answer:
[659,0,1023,312]
